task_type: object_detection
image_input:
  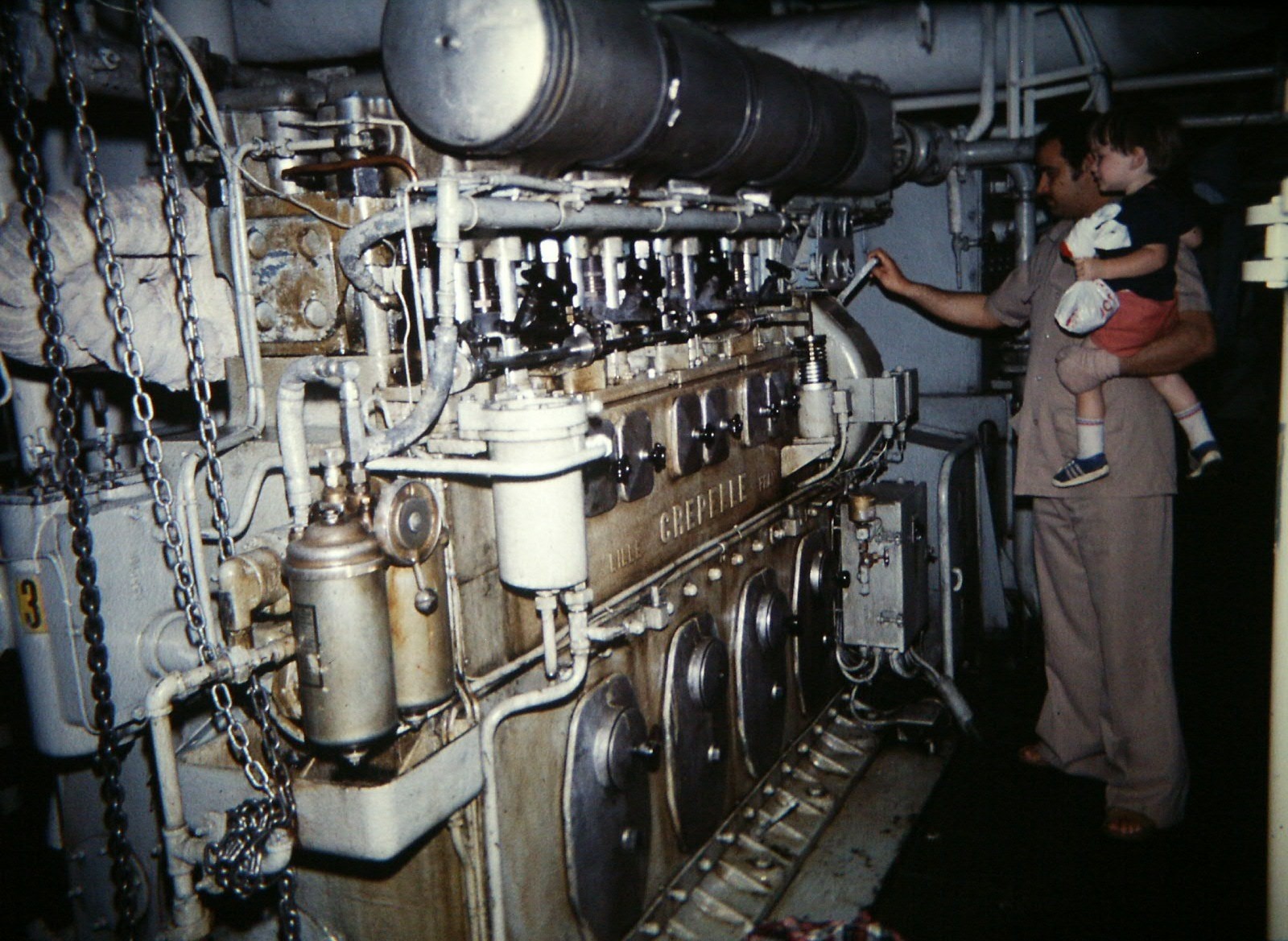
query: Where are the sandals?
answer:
[1017,744,1051,767]
[1104,807,1156,842]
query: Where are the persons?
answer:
[866,110,1219,839]
[1051,108,1223,488]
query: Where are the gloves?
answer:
[1057,345,1120,394]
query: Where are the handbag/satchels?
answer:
[1054,204,1131,334]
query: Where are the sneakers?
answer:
[1186,442,1222,479]
[1052,453,1111,487]
